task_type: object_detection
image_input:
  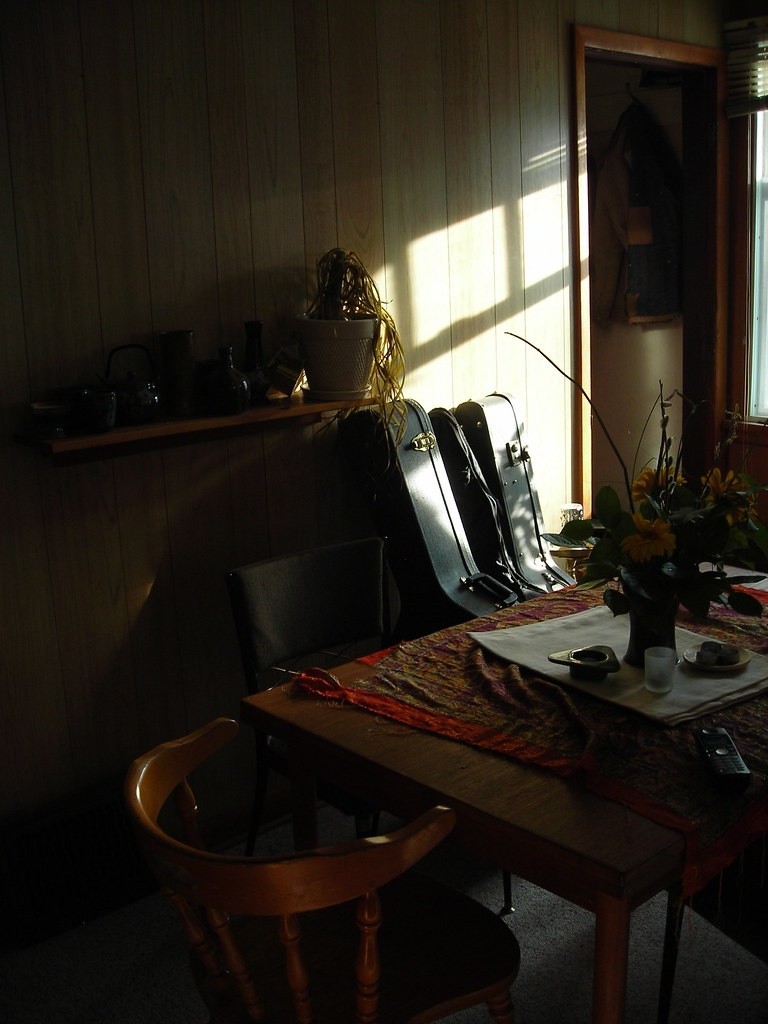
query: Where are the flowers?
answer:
[504,329,768,619]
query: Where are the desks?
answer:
[240,560,768,1023]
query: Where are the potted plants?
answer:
[297,246,410,478]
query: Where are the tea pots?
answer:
[99,344,165,425]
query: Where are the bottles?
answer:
[166,329,200,418]
[198,343,251,418]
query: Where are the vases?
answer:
[620,571,684,667]
[236,320,274,406]
[209,345,251,418]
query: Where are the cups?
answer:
[30,399,72,438]
[50,385,117,433]
[644,646,677,693]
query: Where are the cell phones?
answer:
[692,727,750,784]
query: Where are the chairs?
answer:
[220,538,395,845]
[654,836,768,1024]
[123,717,522,1024]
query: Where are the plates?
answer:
[683,642,751,672]
